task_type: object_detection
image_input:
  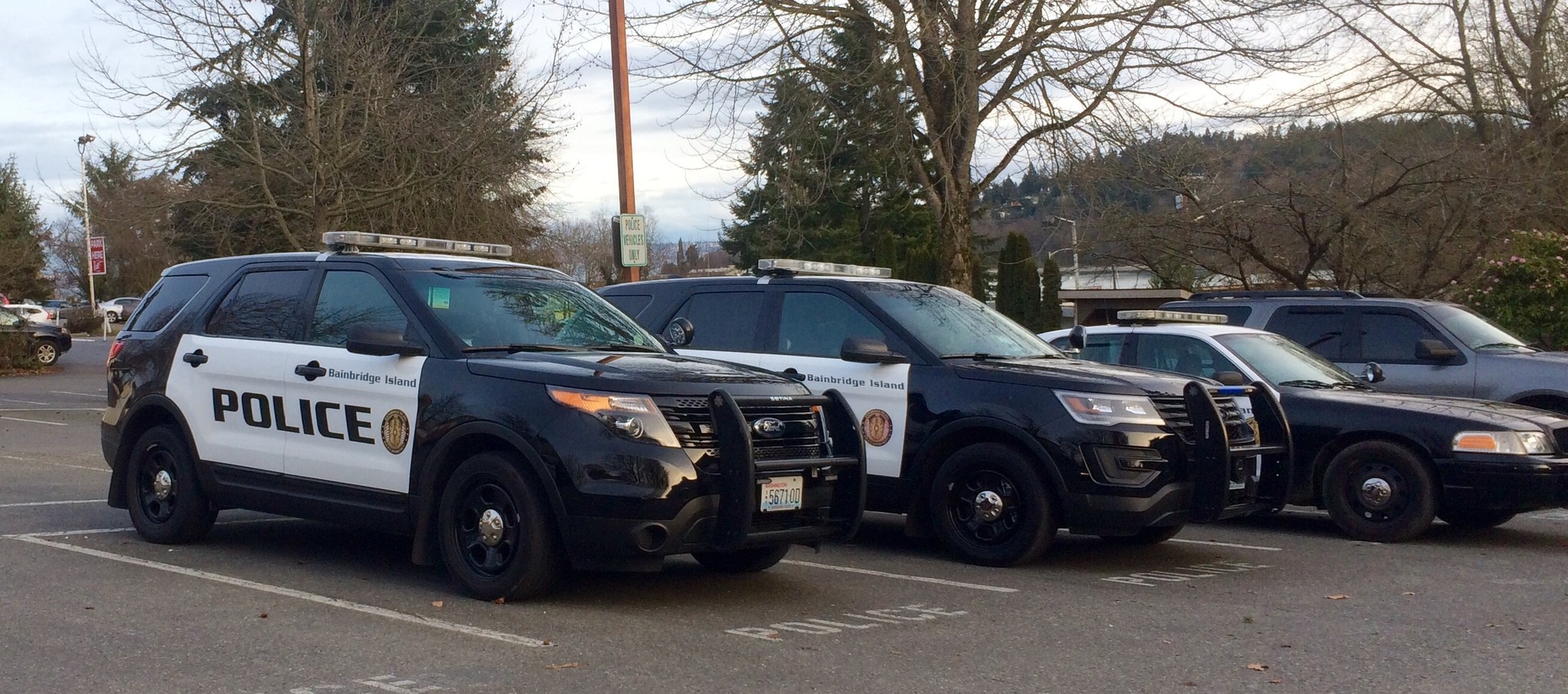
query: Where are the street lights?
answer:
[1042,215,1079,289]
[76,133,97,319]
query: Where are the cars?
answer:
[0,305,72,366]
[1038,309,1568,542]
[95,297,141,324]
[41,299,71,319]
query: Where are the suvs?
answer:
[0,302,52,325]
[100,231,872,604]
[1157,287,1568,418]
[557,259,1288,568]
